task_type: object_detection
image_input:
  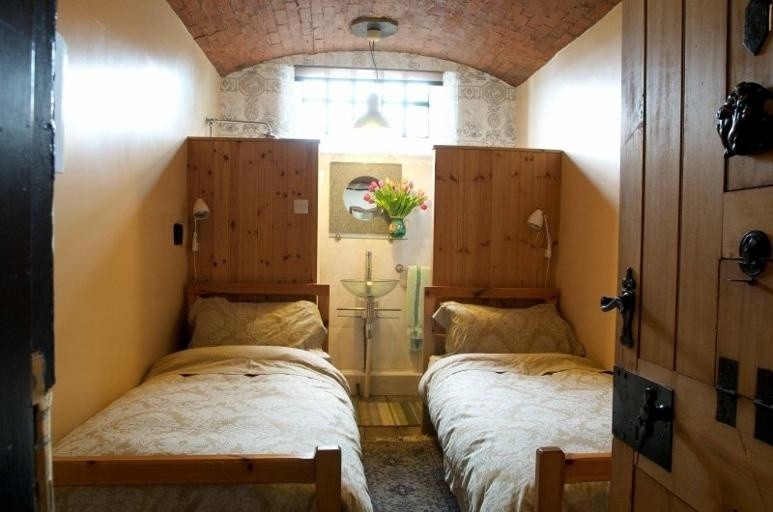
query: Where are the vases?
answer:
[388,219,406,238]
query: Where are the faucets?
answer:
[365,249,372,279]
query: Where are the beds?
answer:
[54,283,372,512]
[422,284,611,511]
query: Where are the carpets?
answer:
[352,398,423,427]
[359,438,461,512]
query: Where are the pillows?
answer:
[434,300,584,355]
[186,295,327,356]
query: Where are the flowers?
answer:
[364,177,428,217]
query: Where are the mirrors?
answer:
[326,161,405,233]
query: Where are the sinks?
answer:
[342,279,400,297]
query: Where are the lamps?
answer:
[191,197,210,282]
[354,30,388,128]
[527,209,552,257]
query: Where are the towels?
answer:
[405,265,432,352]
